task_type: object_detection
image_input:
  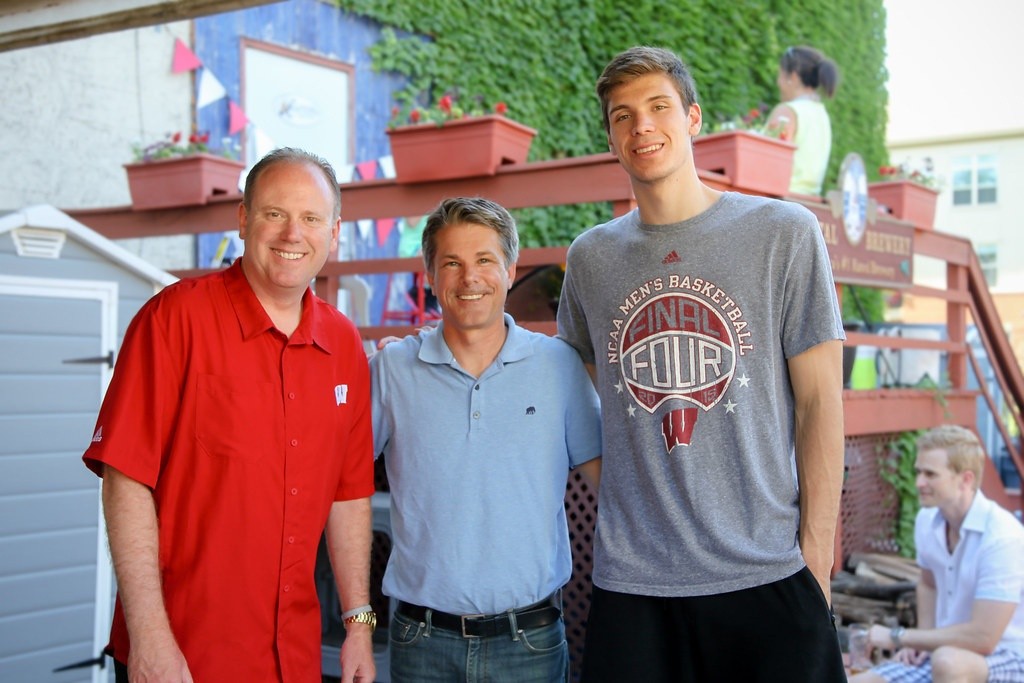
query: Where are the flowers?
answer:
[388,95,507,129]
[876,164,934,188]
[700,108,790,140]
[131,129,212,160]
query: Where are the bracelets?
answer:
[341,605,373,621]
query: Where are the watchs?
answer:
[890,627,903,648]
[343,612,376,632]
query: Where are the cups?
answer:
[847,622,871,672]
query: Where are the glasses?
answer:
[786,46,796,64]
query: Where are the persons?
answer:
[370,197,603,683]
[767,46,837,196]
[375,46,849,683]
[847,424,1024,683]
[81,150,376,683]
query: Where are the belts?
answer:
[397,600,561,638]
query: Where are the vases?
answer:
[122,154,246,211]
[693,130,800,195]
[867,181,939,231]
[387,114,537,185]
